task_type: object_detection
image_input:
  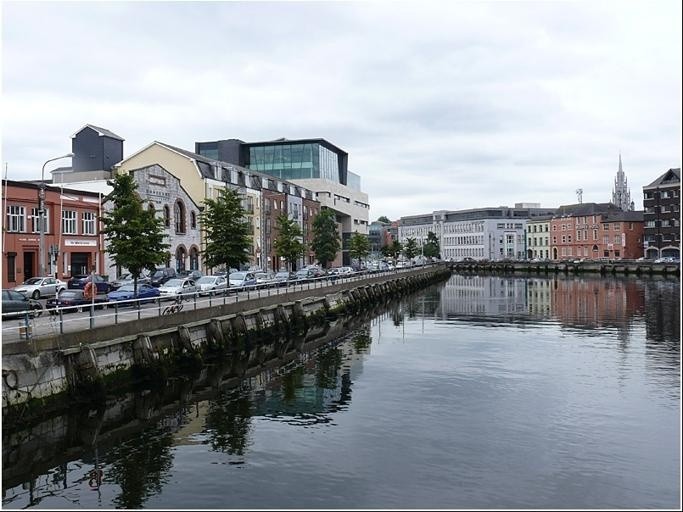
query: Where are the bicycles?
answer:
[161,298,184,316]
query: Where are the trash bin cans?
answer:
[19,320,32,339]
[101,276,108,281]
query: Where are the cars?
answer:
[2,251,457,323]
[274,271,298,287]
[296,255,453,280]
[106,283,160,309]
[145,266,276,301]
[45,289,107,316]
[13,276,68,300]
[67,274,112,294]
[110,272,152,291]
[2,288,43,321]
[461,255,679,265]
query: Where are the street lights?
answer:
[35,152,75,280]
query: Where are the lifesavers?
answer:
[84,283,97,300]
